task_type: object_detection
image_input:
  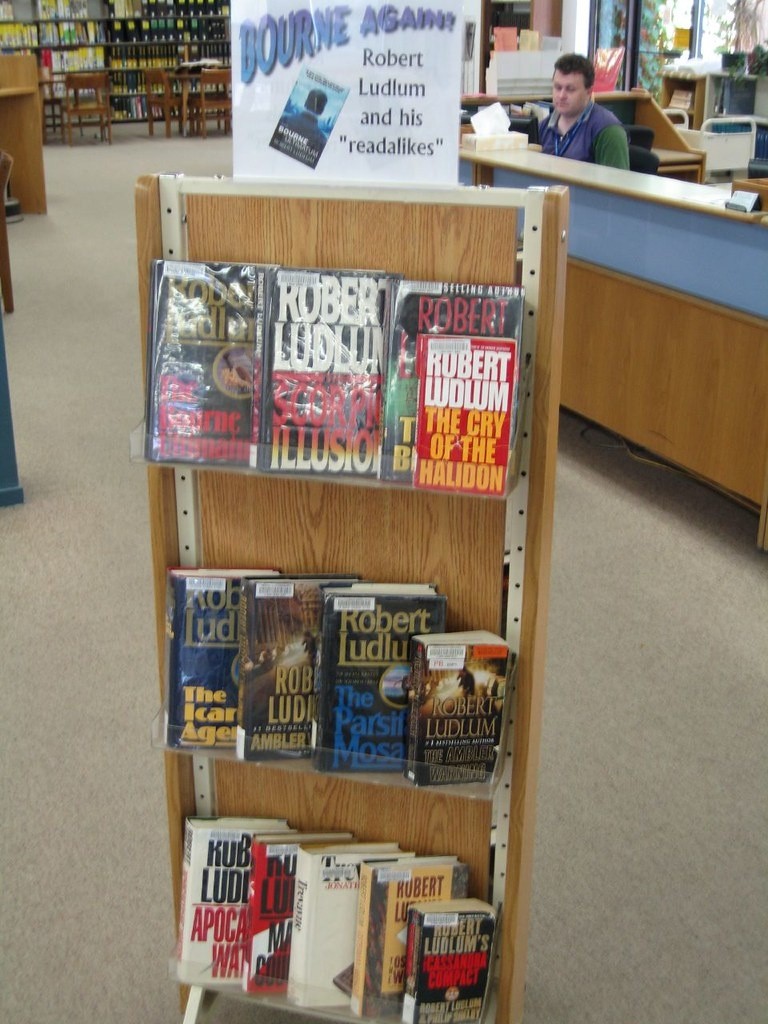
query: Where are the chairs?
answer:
[38,65,73,142]
[187,66,231,139]
[59,70,111,147]
[145,67,190,139]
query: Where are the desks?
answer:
[166,74,232,138]
[468,150,768,549]
[459,140,543,186]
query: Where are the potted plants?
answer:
[747,44,768,81]
[721,53,746,73]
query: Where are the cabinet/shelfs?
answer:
[486,50,575,95]
[460,88,706,185]
[130,177,569,1024]
[654,70,708,131]
[703,71,768,121]
[0,0,231,127]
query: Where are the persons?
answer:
[539,54,630,170]
[270,89,327,168]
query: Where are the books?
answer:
[179,817,495,1024]
[142,258,524,496]
[166,566,510,786]
[0,0,232,123]
